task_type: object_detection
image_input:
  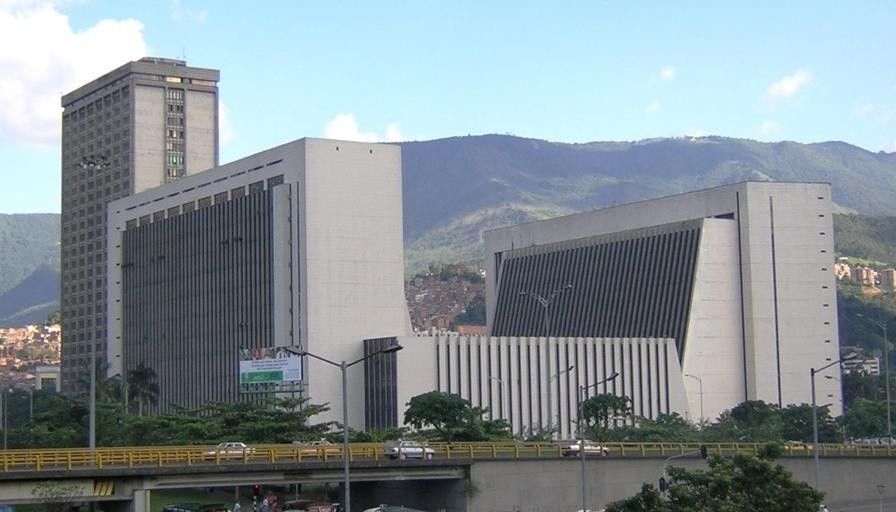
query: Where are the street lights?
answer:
[810,351,856,505]
[517,284,572,441]
[277,341,405,511]
[685,373,704,443]
[576,371,619,511]
[486,377,512,442]
[856,311,892,437]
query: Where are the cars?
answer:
[850,438,896,449]
[779,441,812,450]
[162,500,418,512]
[563,440,609,456]
[200,441,254,461]
[383,440,434,460]
[291,440,340,459]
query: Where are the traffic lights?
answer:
[659,477,665,492]
[700,445,706,458]
